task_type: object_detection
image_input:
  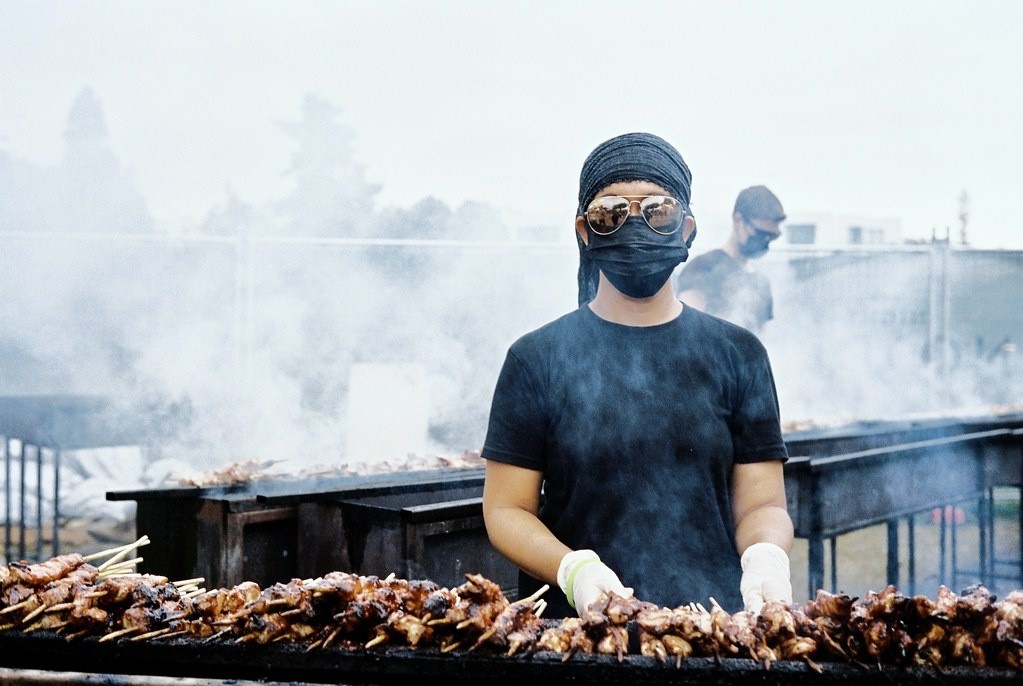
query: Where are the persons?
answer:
[481,133,794,620]
[593,204,622,229]
[676,184,787,343]
[643,206,653,222]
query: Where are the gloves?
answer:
[740,542,793,618]
[557,549,635,618]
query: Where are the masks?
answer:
[585,218,688,298]
[735,223,770,259]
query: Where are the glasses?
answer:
[584,195,687,235]
[744,215,782,241]
